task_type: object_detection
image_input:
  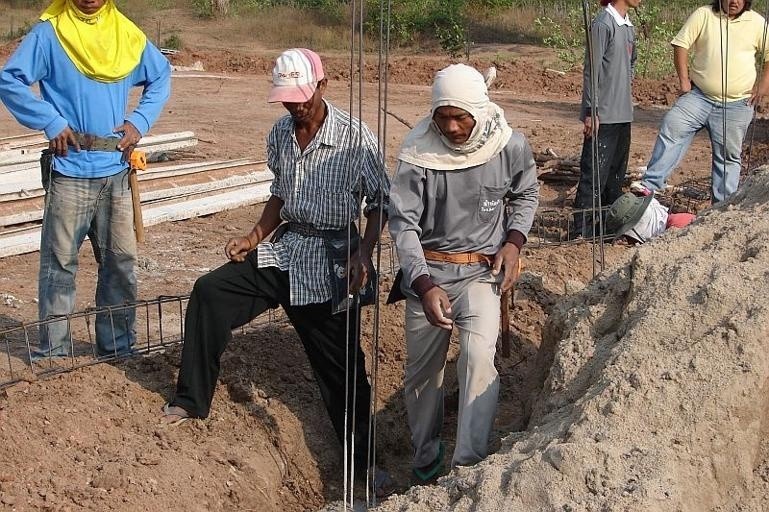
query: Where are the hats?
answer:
[268,48,322,103]
[606,193,655,240]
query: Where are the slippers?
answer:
[408,441,446,489]
[369,467,398,498]
[159,401,188,428]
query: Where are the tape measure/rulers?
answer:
[129,150,147,171]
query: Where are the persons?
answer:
[158,46,398,498]
[0,0,171,364]
[387,61,542,488]
[574,0,642,243]
[630,0,769,205]
